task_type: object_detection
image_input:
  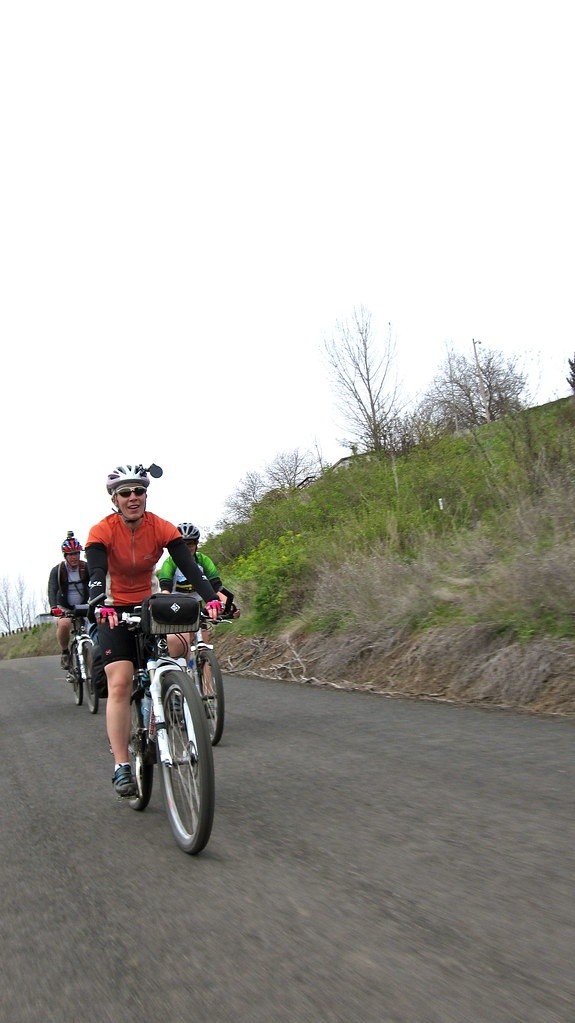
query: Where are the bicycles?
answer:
[86,592,233,854]
[178,586,240,745]
[52,604,100,714]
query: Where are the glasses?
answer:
[118,487,145,498]
[184,540,196,546]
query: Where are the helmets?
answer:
[61,539,82,553]
[176,522,201,539]
[107,464,150,495]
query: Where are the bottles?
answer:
[143,691,153,728]
[187,660,193,674]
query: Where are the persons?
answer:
[47,463,239,796]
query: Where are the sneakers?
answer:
[61,652,73,670]
[112,764,138,796]
